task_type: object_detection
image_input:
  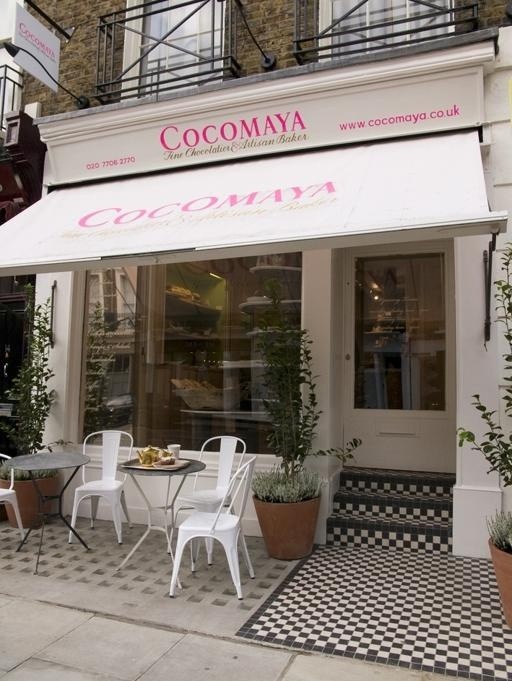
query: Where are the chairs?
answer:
[169,454,259,601]
[166,434,247,566]
[0,454,27,545]
[68,429,135,545]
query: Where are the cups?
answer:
[167,444,182,460]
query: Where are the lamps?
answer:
[3,40,90,110]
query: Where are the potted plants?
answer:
[1,283,74,529]
[454,239,512,630]
[251,269,364,560]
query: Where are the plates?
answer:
[151,459,190,469]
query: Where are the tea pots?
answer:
[136,443,159,465]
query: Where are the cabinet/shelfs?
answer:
[239,265,302,409]
[164,295,224,387]
